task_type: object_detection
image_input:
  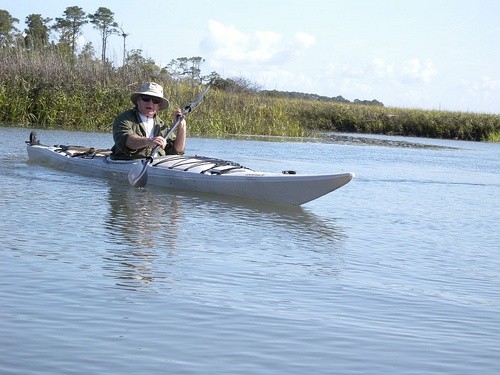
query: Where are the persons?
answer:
[111,83,186,158]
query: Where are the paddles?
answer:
[127,75,219,189]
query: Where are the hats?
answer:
[131,81,170,109]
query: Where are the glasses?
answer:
[140,94,161,104]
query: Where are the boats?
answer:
[26,131,352,208]
